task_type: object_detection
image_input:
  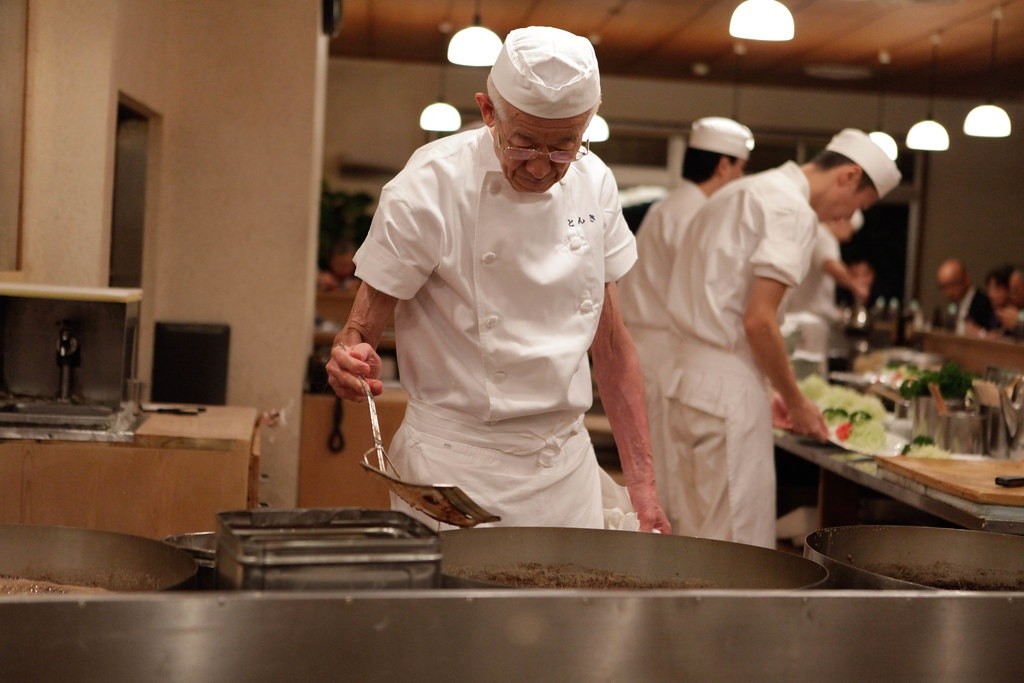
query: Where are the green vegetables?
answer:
[900,434,933,455]
[823,408,871,423]
[884,357,977,399]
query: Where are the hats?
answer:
[825,128,902,200]
[688,116,755,162]
[850,208,865,232]
[491,25,601,119]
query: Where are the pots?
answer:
[1,522,1024,599]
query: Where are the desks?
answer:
[773,431,1023,537]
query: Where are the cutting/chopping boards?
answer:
[876,456,1024,506]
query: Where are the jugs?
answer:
[996,372,1024,459]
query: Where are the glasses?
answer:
[493,112,590,164]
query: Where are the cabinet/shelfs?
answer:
[0,406,260,534]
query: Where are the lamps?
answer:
[962,7,1011,137]
[417,22,463,132]
[868,52,899,159]
[447,0,503,67]
[906,35,951,151]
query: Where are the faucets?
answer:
[53,318,82,402]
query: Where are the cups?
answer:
[934,404,994,456]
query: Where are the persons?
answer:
[662,128,903,551]
[616,114,755,503]
[786,205,871,351]
[927,257,1024,342]
[324,26,670,533]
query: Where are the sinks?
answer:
[0,417,112,432]
[1,404,114,415]
